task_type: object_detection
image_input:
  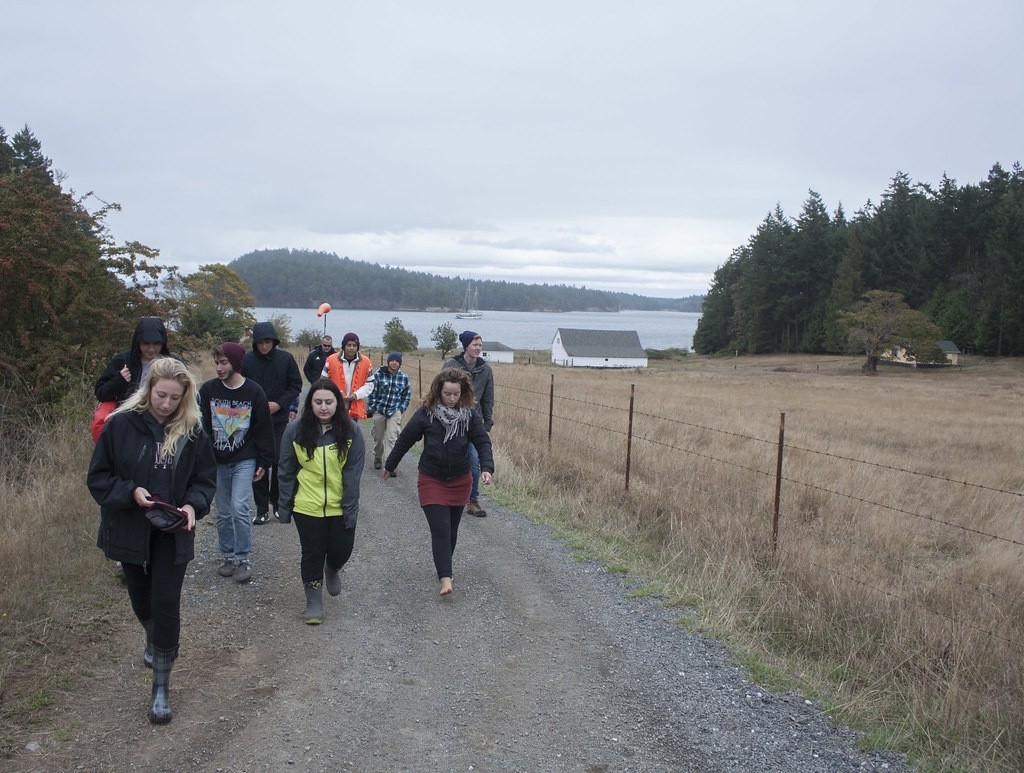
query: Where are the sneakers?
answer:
[467,496,487,518]
[389,471,398,477]
[272,506,281,520]
[218,560,236,577]
[235,560,253,581]
[252,512,271,525]
[374,458,383,469]
[113,561,123,578]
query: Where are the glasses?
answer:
[323,344,333,348]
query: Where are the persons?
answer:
[95,317,184,421]
[87,357,218,722]
[320,333,376,423]
[382,367,495,595]
[243,322,303,525]
[445,330,494,518]
[302,335,337,384]
[279,378,367,626]
[368,353,411,476]
[197,341,272,583]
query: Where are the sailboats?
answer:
[456,281,483,319]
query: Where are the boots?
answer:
[326,562,342,596]
[304,580,325,624]
[138,615,155,668]
[148,642,180,727]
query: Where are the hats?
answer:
[388,352,402,366]
[341,333,360,351]
[212,342,246,373]
[459,331,482,352]
